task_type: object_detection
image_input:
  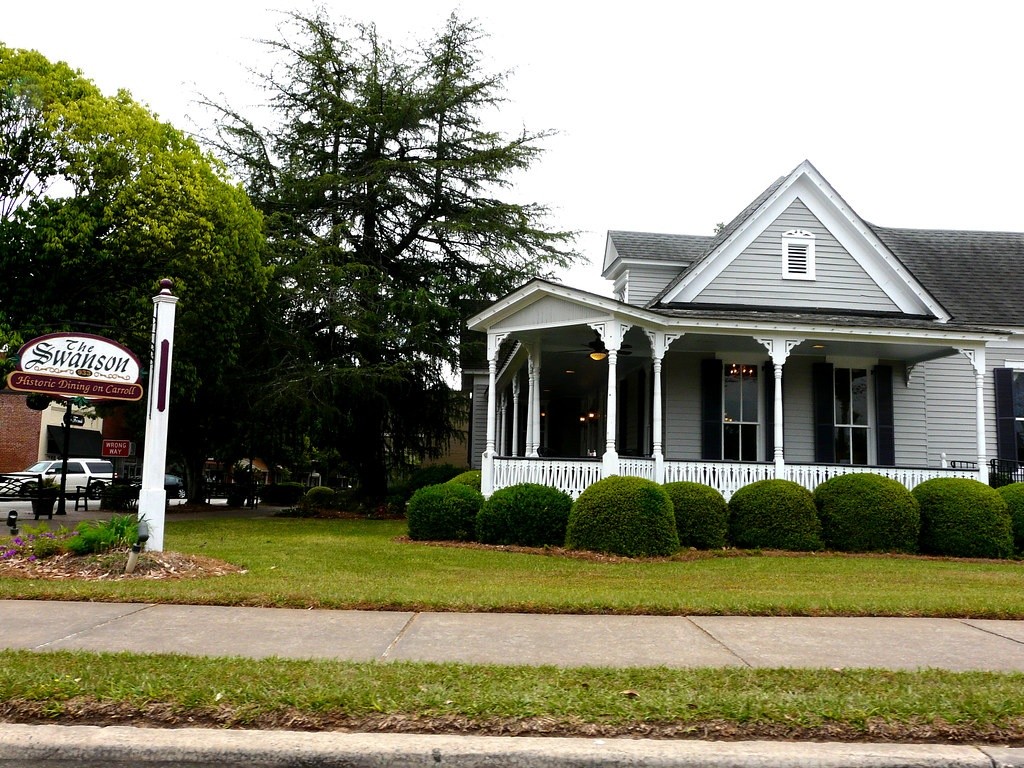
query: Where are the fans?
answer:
[555,330,633,359]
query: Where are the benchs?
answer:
[72,475,141,512]
[205,482,260,509]
[0,474,65,519]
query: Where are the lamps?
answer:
[588,331,607,361]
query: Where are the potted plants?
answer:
[25,478,62,516]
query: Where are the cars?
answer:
[126,472,187,499]
[0,458,118,501]
